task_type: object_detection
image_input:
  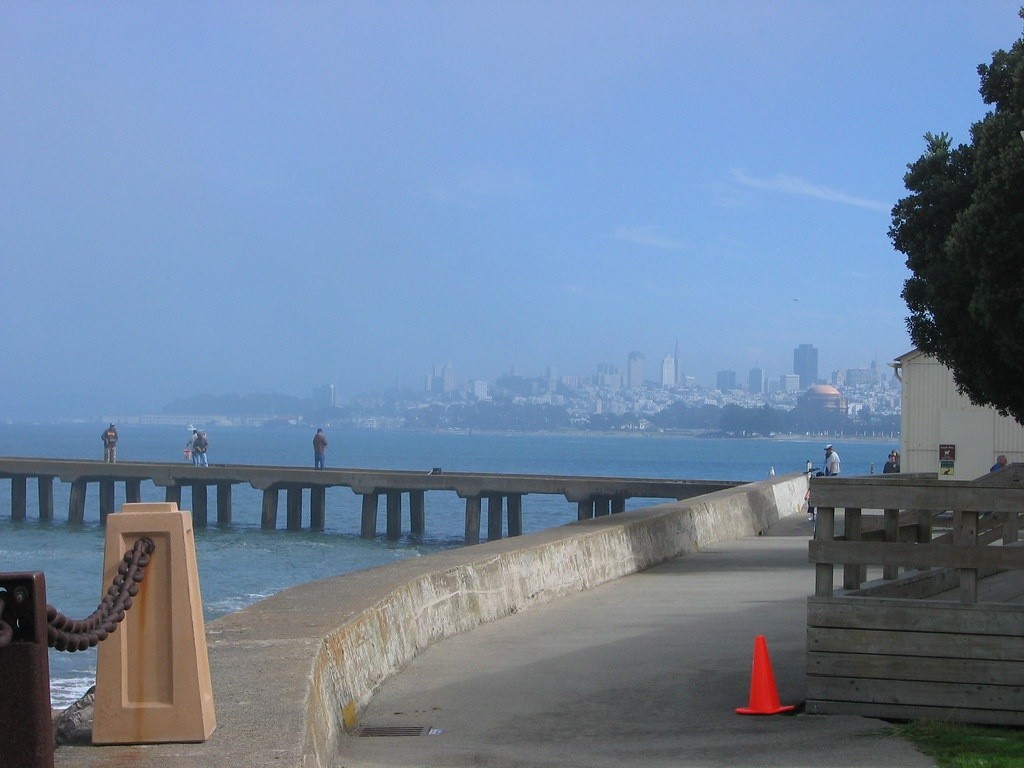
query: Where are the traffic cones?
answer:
[734,634,796,716]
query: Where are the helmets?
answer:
[888,450,898,460]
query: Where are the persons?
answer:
[101,422,118,464]
[312,427,329,470]
[823,442,841,476]
[982,454,1008,519]
[884,450,900,473]
[185,429,209,467]
[804,472,826,522]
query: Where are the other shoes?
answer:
[808,512,814,522]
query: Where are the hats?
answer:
[824,444,832,450]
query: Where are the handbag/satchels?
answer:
[184,450,192,459]
